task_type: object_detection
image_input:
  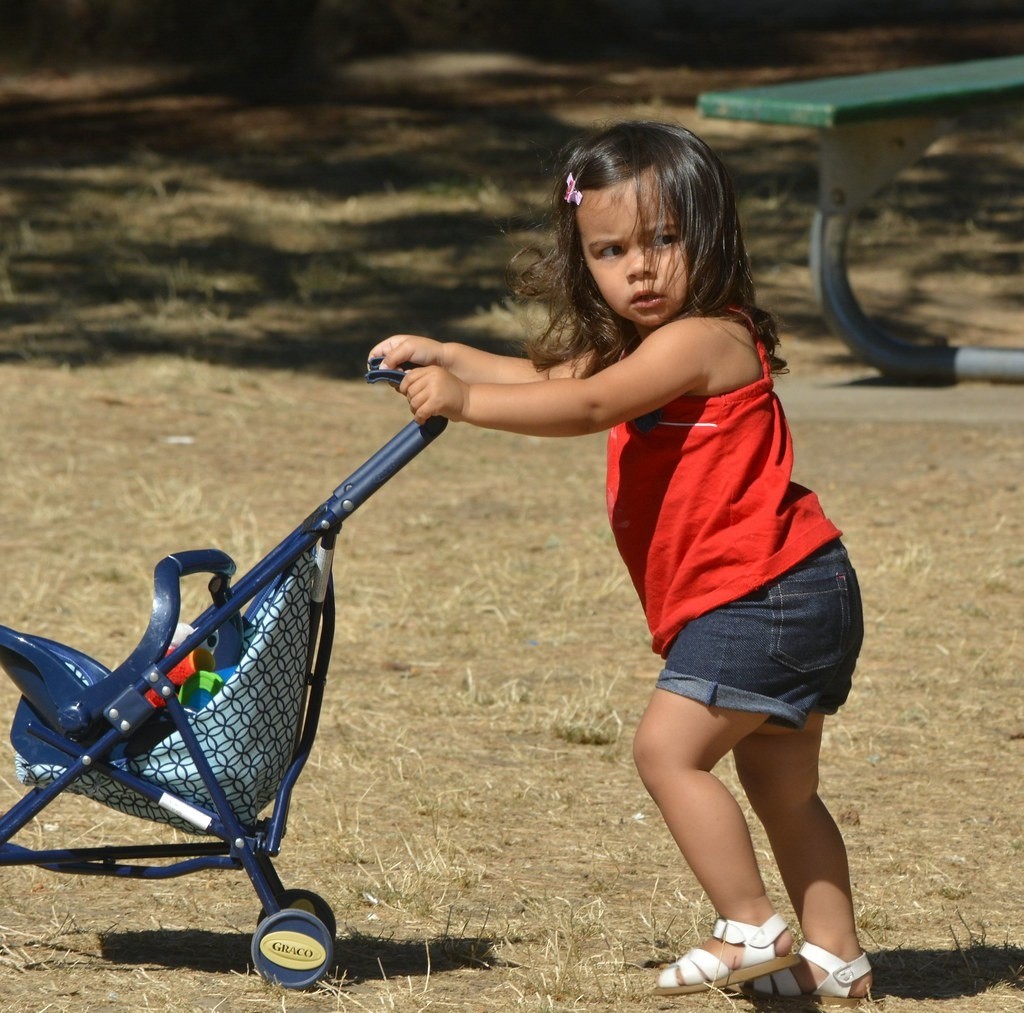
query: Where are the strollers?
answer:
[1,352,449,995]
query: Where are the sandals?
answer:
[730,941,872,1005]
[655,915,799,996]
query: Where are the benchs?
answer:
[699,52,1023,383]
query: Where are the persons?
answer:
[367,120,872,1006]
